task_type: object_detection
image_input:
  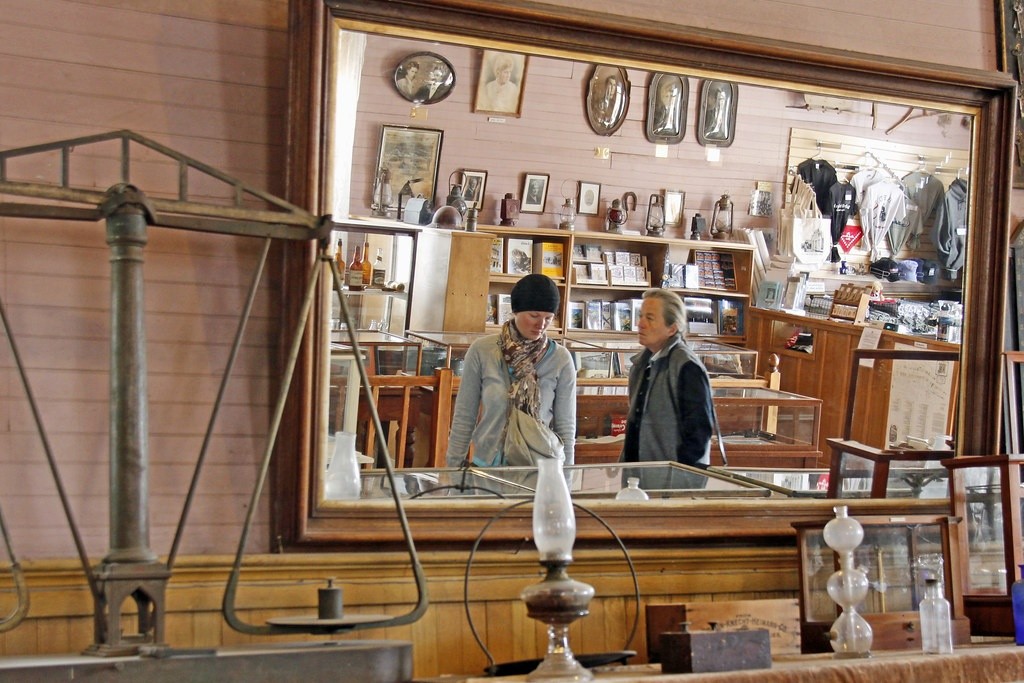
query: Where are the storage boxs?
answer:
[645,597,801,674]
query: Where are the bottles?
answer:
[333,238,345,290]
[1011,564,1024,646]
[917,552,952,655]
[371,247,385,288]
[349,246,363,291]
[361,242,371,285]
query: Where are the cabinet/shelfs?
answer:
[327,216,823,470]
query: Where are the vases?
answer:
[1011,565,1024,645]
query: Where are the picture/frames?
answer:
[367,38,740,226]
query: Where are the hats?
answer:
[511,274,560,316]
[869,257,940,285]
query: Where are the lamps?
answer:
[711,191,735,240]
[447,170,469,228]
[644,193,665,238]
[519,458,597,682]
[559,177,579,230]
[823,504,873,657]
[372,167,394,217]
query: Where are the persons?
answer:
[464,179,480,201]
[525,181,544,205]
[616,289,716,490]
[655,83,679,138]
[592,75,620,128]
[705,87,730,142]
[482,55,520,112]
[446,274,576,494]
[396,61,451,102]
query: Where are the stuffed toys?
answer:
[866,280,885,302]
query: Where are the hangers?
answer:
[803,107,968,189]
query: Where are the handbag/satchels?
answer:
[504,409,566,466]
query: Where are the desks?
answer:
[430,637,1024,683]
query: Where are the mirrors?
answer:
[277,2,1020,559]
